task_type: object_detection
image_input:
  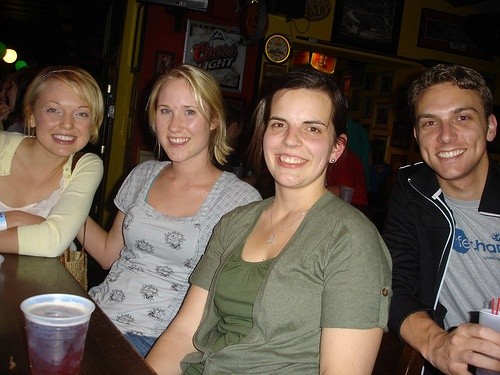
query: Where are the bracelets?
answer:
[0,212,7,231]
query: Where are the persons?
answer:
[383,65,500,375]
[145,69,392,375]
[0,67,41,134]
[75,66,262,360]
[218,90,369,216]
[0,65,104,259]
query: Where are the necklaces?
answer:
[267,203,309,246]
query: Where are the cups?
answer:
[340,186,354,205]
[20,293,96,375]
[475,307,500,375]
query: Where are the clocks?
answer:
[263,33,292,65]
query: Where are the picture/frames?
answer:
[153,49,176,78]
